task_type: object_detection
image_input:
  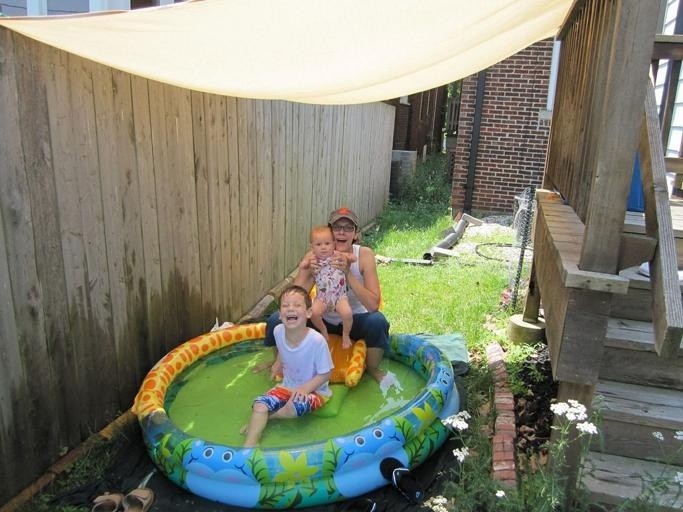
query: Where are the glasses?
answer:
[331,225,354,232]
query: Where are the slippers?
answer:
[380,458,423,506]
[92,492,124,512]
[122,488,154,512]
[334,495,387,512]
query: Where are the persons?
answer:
[299,225,358,349]
[264,208,390,386]
[240,286,336,448]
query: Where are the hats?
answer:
[328,208,358,227]
